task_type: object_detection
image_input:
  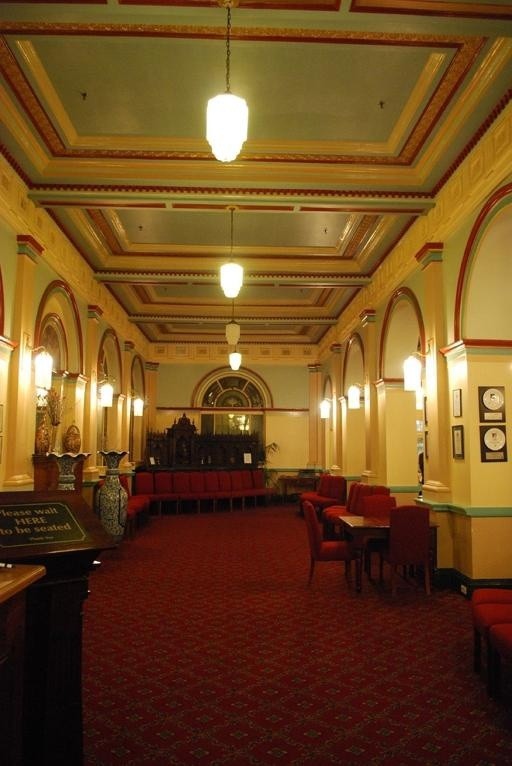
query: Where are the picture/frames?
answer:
[453,389,462,417]
[452,425,464,458]
[424,431,429,459]
[424,397,428,425]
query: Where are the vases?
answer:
[97,450,130,545]
[45,451,92,490]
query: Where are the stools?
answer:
[472,588,512,682]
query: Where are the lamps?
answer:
[19,332,144,418]
[318,337,432,420]
[205,1,250,370]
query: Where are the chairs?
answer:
[299,474,431,599]
[94,466,276,541]
